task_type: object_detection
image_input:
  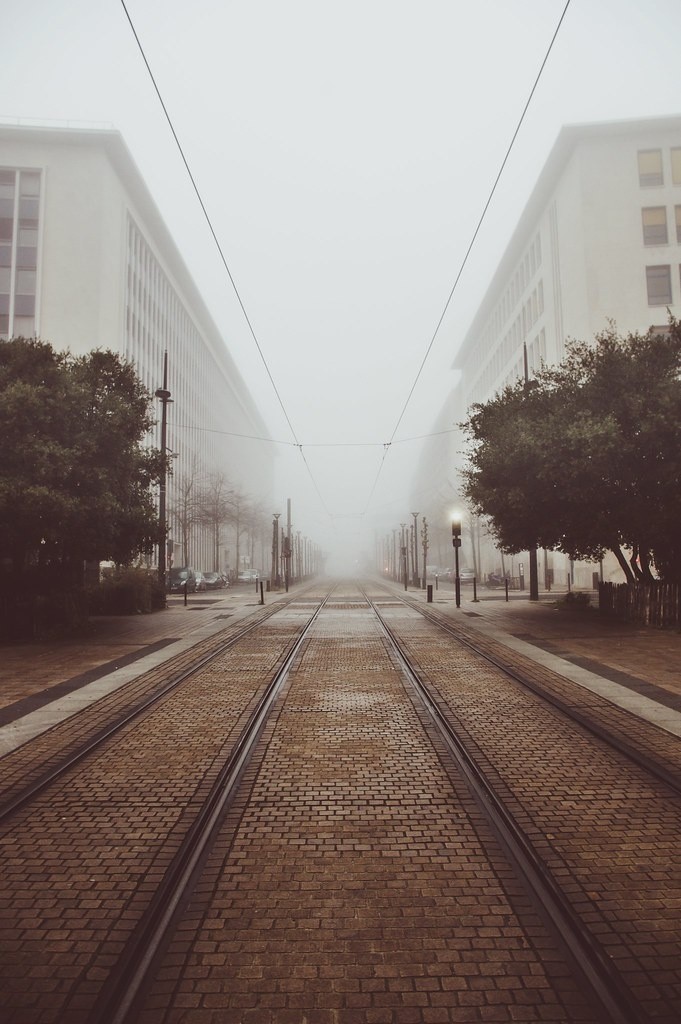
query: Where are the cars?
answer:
[163,566,270,594]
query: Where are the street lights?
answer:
[153,349,173,616]
[451,509,466,608]
